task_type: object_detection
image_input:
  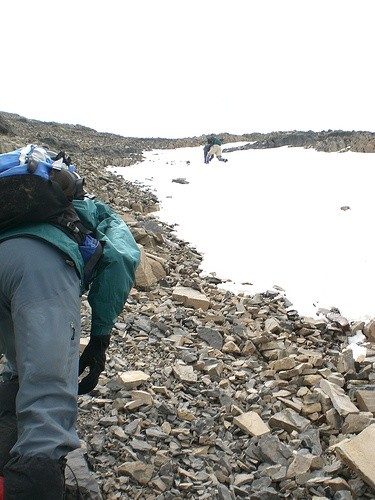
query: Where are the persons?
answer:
[205,134,228,164]
[1,144,142,500]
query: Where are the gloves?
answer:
[78,335,107,395]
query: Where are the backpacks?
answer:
[0,144,84,238]
